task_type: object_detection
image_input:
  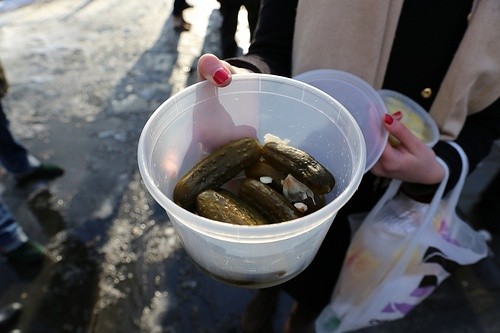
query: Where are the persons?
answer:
[197,0,500,332]
[0,56,66,278]
[173,0,263,58]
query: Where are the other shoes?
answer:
[12,239,50,269]
[15,158,65,186]
[175,24,190,31]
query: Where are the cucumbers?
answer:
[173,137,336,226]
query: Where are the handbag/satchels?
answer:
[314,137,489,333]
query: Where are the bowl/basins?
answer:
[137,74,366,289]
[371,90,439,148]
